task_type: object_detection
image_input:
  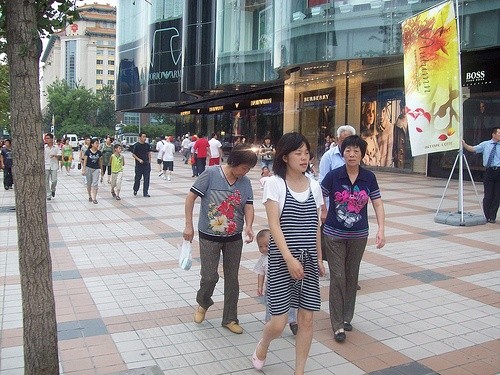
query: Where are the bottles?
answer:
[83,175,87,183]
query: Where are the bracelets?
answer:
[317,258,324,263]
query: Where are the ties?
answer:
[487,143,497,168]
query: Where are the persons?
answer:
[321,135,386,341]
[251,132,323,375]
[43,122,360,335]
[0,136,19,190]
[360,100,412,169]
[462,128,500,223]
[473,97,493,167]
[131,132,150,197]
[182,144,258,335]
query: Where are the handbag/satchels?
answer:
[179,239,192,270]
[64,156,68,161]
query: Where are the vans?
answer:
[63,134,79,151]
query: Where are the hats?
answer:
[186,134,189,137]
[192,136,197,141]
[169,136,173,142]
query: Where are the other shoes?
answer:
[251,338,267,370]
[224,320,243,334]
[344,322,352,331]
[145,194,150,197]
[289,322,298,335]
[488,217,496,223]
[111,191,116,196]
[93,200,98,204]
[52,192,55,197]
[334,330,346,342]
[134,190,137,195]
[194,304,206,323]
[116,196,120,200]
[89,197,92,202]
[47,198,51,200]
[159,172,171,181]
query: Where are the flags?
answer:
[399,0,462,156]
